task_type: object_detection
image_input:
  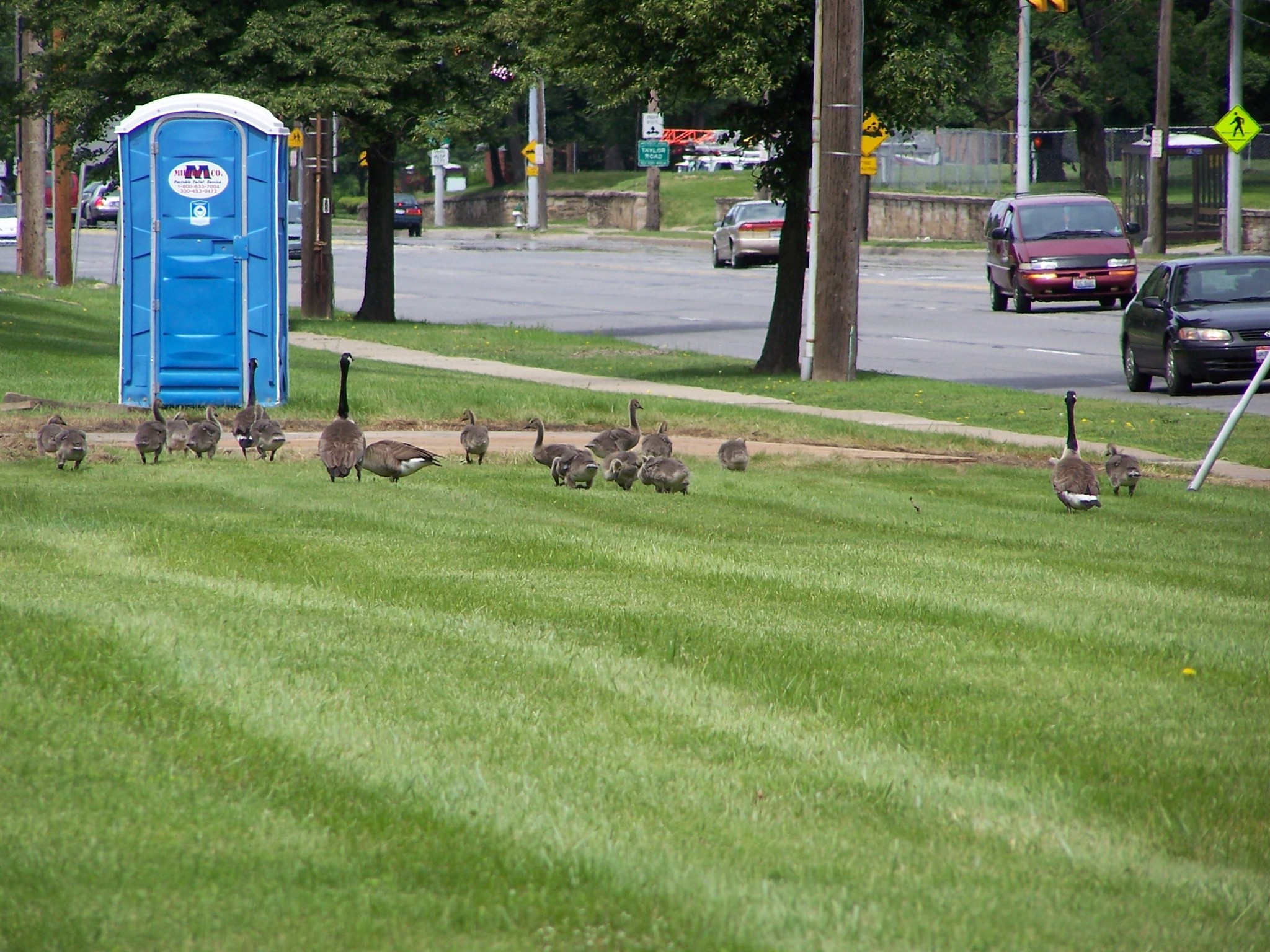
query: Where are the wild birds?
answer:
[549,449,597,489]
[358,441,446,483]
[586,398,645,461]
[521,418,578,468]
[601,452,643,491]
[36,414,88,472]
[133,399,221,464]
[1106,440,1144,497]
[1052,390,1103,512]
[459,408,490,465]
[318,353,369,483]
[640,422,690,497]
[230,358,286,463]
[718,434,750,472]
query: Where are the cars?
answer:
[80,181,122,225]
[710,200,812,268]
[393,193,423,236]
[0,180,19,245]
[1120,255,1270,397]
[684,128,771,157]
[287,198,303,259]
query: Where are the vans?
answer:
[44,169,80,214]
[983,190,1142,314]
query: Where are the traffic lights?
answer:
[1026,0,1073,14]
